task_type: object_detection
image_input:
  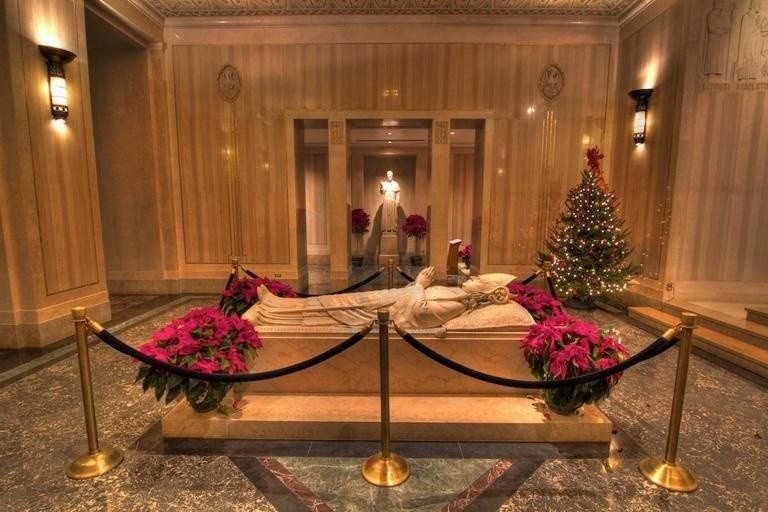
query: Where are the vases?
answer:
[351,255,363,267]
[181,379,230,411]
[543,380,587,415]
[410,251,421,265]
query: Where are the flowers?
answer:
[403,215,429,255]
[508,281,568,322]
[349,209,371,256]
[128,307,262,406]
[456,245,473,267]
[217,273,295,313]
[519,317,629,400]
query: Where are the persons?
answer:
[380,171,401,234]
[253,264,519,328]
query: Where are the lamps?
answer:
[627,89,653,145]
[39,41,80,121]
